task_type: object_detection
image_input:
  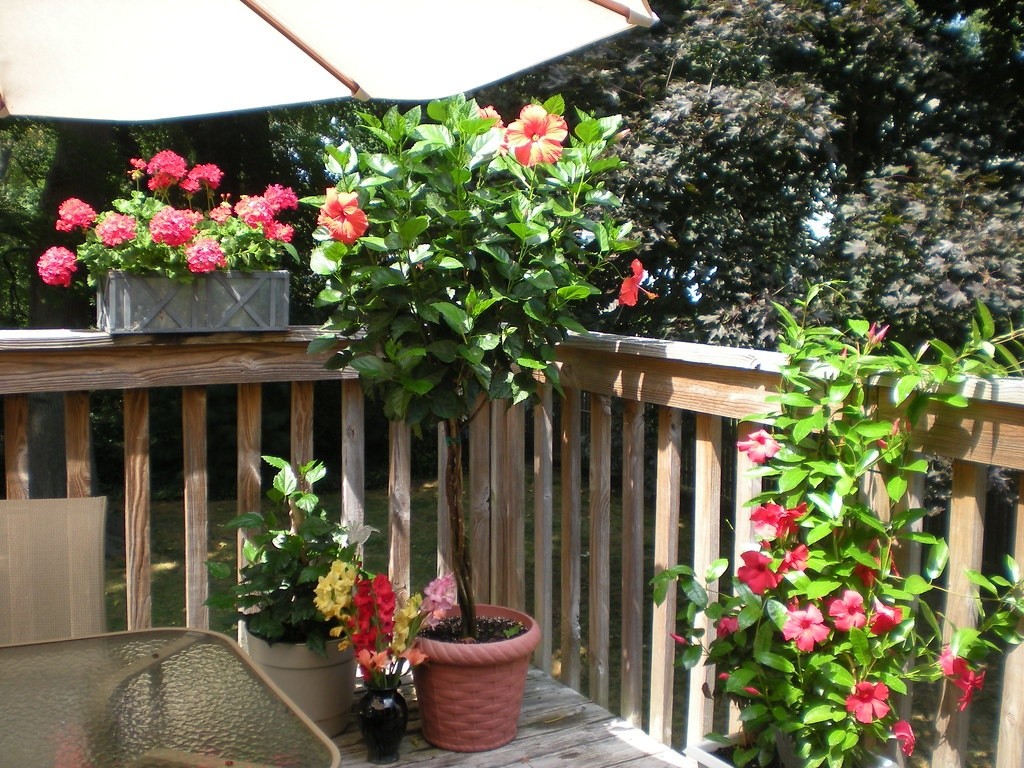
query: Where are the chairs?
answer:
[0,495,108,647]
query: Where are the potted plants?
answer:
[200,455,378,738]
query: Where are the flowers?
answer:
[298,91,658,624]
[657,302,1024,768]
[36,150,298,288]
[312,558,458,683]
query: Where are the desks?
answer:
[0,626,342,768]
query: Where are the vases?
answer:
[409,604,542,752]
[682,731,894,768]
[94,268,290,333]
[356,677,408,764]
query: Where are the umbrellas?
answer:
[0,0,660,122]
